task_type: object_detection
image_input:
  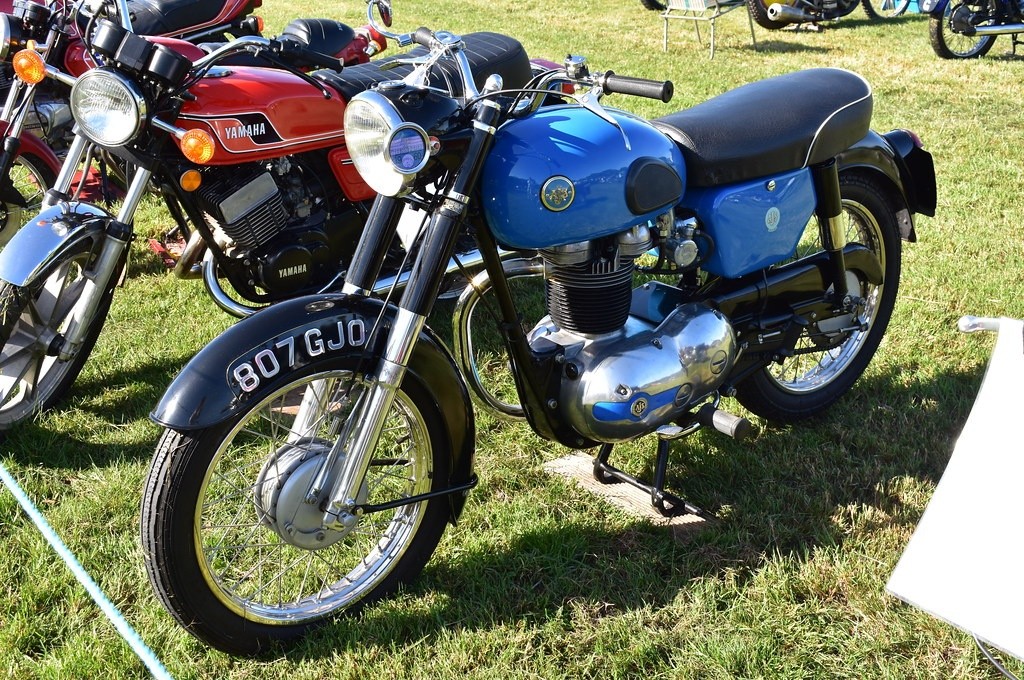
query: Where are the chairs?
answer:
[659,0,759,61]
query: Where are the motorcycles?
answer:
[0,0,577,439]
[746,0,910,34]
[140,0,937,660]
[0,0,265,249]
[920,0,1024,59]
